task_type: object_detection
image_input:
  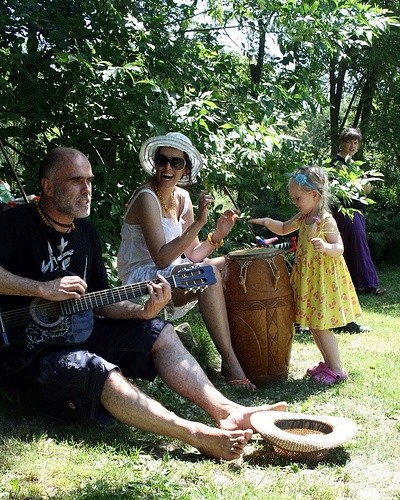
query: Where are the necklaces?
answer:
[153,179,176,218]
[35,200,75,234]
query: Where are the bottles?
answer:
[0,183,17,206]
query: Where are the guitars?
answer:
[0,263,217,356]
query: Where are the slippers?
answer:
[313,367,348,385]
[220,375,257,393]
[307,362,328,376]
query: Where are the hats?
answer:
[139,132,203,187]
[250,411,358,462]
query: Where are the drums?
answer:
[222,246,297,385]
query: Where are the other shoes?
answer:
[365,287,386,294]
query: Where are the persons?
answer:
[247,166,362,386]
[327,127,386,297]
[117,131,256,391]
[0,147,288,461]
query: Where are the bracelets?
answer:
[208,231,224,248]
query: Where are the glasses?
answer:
[153,154,186,170]
